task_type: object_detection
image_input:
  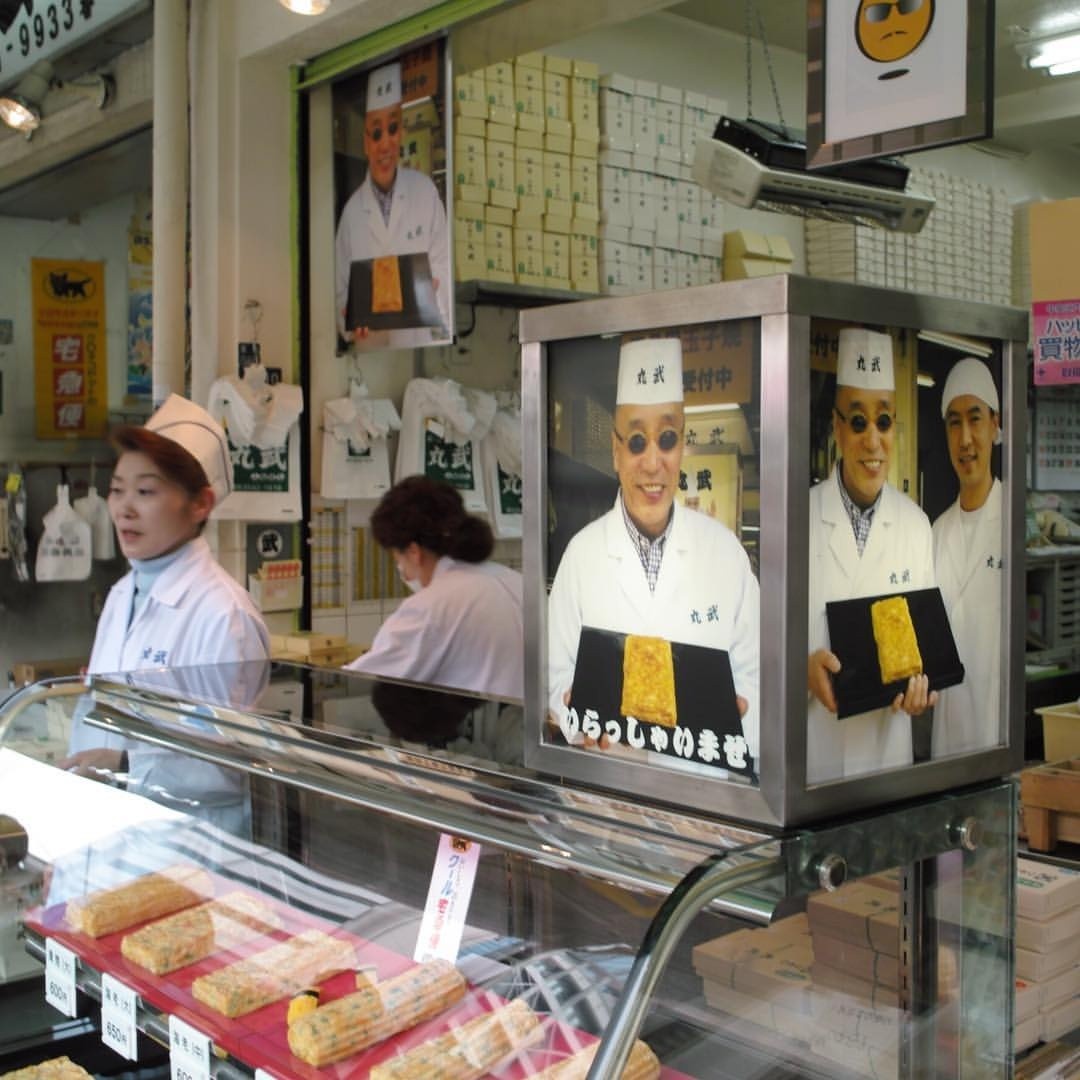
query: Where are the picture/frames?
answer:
[806,0,996,174]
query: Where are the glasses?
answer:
[614,427,679,452]
[834,405,894,433]
[366,122,400,141]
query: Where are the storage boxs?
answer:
[452,51,795,295]
[806,158,1013,306]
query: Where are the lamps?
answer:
[0,59,114,132]
[1014,30,1080,81]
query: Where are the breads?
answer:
[871,596,922,685]
[0,1055,96,1080]
[620,635,676,728]
[370,254,402,312]
[61,861,662,1080]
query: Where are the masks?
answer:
[396,554,424,592]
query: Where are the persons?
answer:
[326,62,448,352]
[547,328,1008,784]
[62,393,272,847]
[342,474,521,701]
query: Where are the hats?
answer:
[144,393,234,508]
[617,337,684,405]
[837,328,893,390]
[366,64,401,111]
